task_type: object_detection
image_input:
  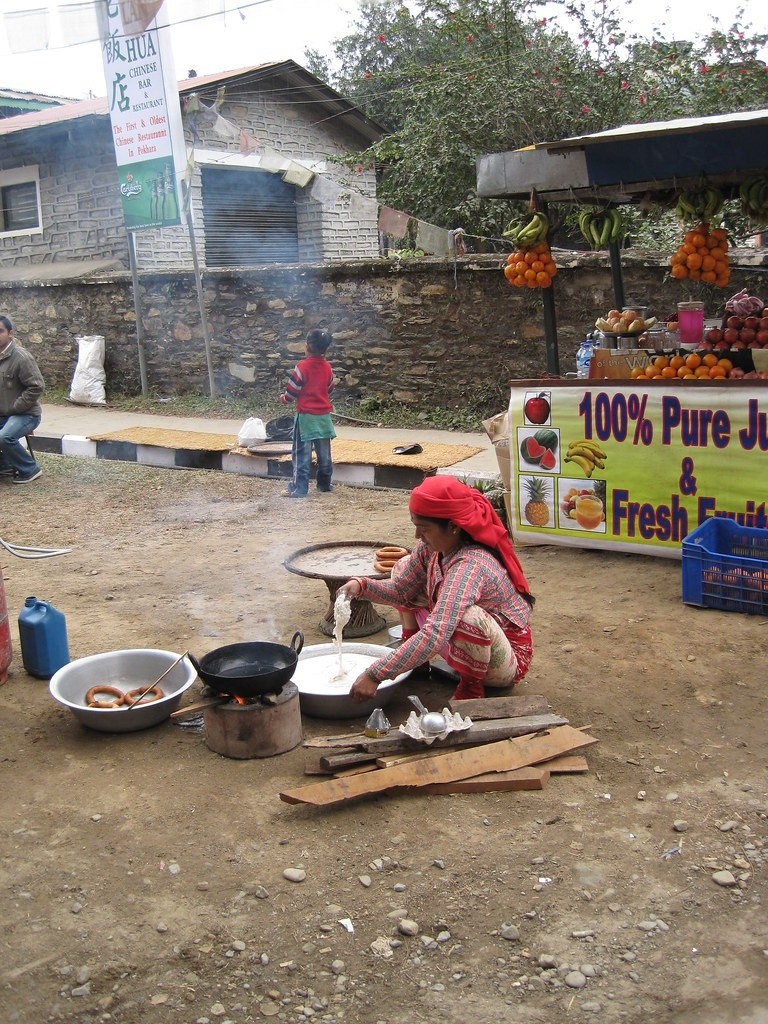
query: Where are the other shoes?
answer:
[406,667,432,680]
[437,697,457,718]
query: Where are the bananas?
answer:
[676,186,724,223]
[564,438,607,478]
[501,211,550,247]
[740,173,768,227]
[580,207,622,251]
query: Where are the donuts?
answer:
[85,684,163,710]
[374,546,408,572]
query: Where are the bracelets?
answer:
[365,668,383,686]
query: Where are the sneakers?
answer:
[280,489,297,498]
[13,468,42,486]
[315,484,333,491]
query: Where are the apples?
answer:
[525,392,550,425]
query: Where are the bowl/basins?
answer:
[46,648,197,733]
[287,642,415,719]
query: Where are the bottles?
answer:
[573,299,705,381]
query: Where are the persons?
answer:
[280,327,338,500]
[336,476,537,705]
[0,314,46,484]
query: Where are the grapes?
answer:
[664,312,678,322]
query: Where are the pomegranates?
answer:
[698,308,768,350]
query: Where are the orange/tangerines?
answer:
[606,310,644,333]
[669,223,731,286]
[502,241,556,288]
[568,488,578,496]
[630,354,733,380]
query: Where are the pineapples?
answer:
[520,476,552,525]
[592,480,606,520]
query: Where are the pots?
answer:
[186,630,305,698]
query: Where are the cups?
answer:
[575,496,604,531]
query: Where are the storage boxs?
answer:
[682,518,768,617]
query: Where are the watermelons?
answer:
[520,430,557,470]
